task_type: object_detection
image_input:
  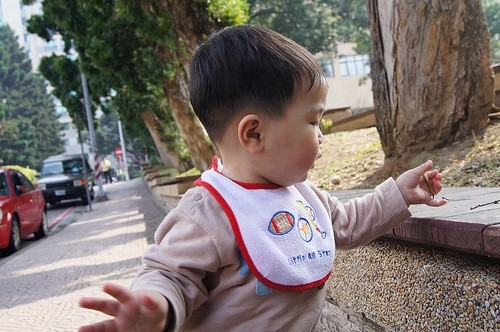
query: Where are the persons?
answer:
[78,25,448,332]
[101,157,112,183]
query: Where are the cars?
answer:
[1,165,37,182]
[0,169,50,253]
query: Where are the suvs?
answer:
[36,154,94,206]
